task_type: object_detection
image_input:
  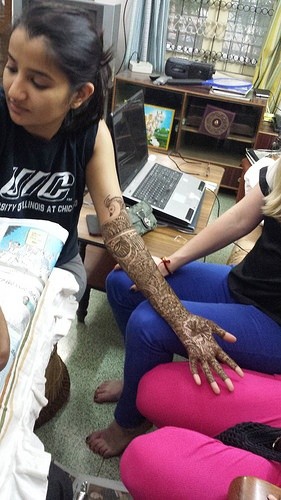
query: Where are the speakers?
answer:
[197,103,236,142]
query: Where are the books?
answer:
[209,70,272,102]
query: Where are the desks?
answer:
[77,151,225,321]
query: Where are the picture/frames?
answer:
[123,100,175,151]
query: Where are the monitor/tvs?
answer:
[11,0,120,88]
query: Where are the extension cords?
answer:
[129,60,153,74]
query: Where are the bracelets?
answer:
[161,256,173,276]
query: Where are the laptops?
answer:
[110,88,205,224]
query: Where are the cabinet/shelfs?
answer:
[111,70,267,203]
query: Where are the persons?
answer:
[86,143,281,458]
[118,359,281,500]
[0,2,245,395]
